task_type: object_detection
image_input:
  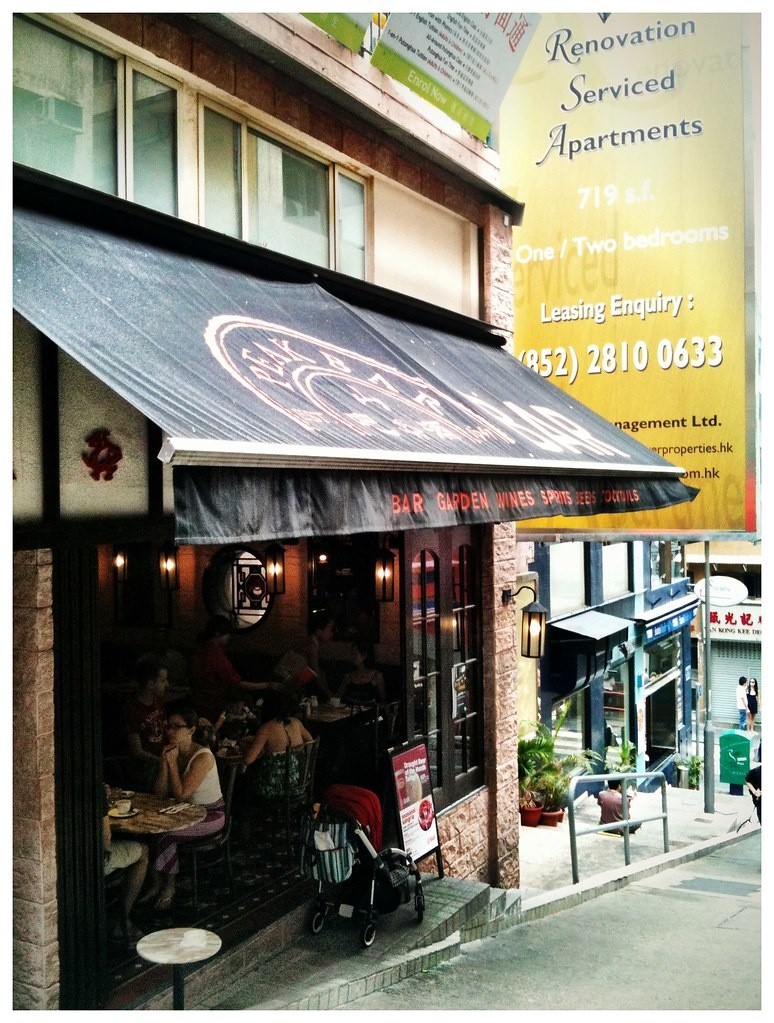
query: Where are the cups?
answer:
[331,698,341,707]
[115,800,132,814]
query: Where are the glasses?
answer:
[166,723,189,730]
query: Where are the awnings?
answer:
[13,208,702,547]
[643,601,701,645]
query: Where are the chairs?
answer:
[176,759,247,912]
[250,733,321,866]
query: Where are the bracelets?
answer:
[268,679,272,689]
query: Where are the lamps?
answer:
[374,532,399,603]
[503,586,548,659]
[158,540,179,592]
[265,540,286,597]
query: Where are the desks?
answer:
[103,785,226,929]
[301,697,374,725]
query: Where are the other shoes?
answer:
[114,919,143,937]
[153,886,175,910]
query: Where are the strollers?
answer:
[296,784,424,950]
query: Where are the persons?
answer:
[745,764,761,826]
[208,688,257,744]
[124,664,183,792]
[353,606,373,639]
[736,676,760,731]
[103,784,150,938]
[597,780,642,835]
[232,686,314,845]
[293,611,335,706]
[136,707,226,912]
[331,639,385,772]
[186,614,286,725]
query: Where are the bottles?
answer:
[311,696,318,706]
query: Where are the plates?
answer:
[108,809,139,817]
[216,748,243,758]
[118,791,135,798]
[325,703,346,708]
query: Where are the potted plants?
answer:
[520,700,606,827]
